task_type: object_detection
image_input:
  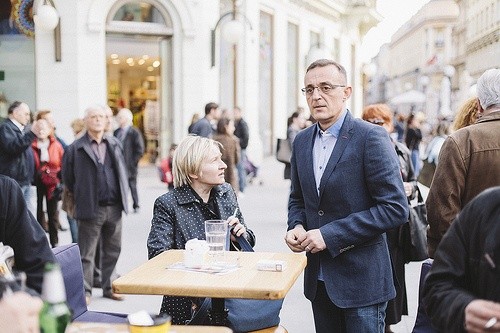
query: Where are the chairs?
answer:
[51,242,130,325]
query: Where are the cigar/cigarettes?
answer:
[233,208,239,220]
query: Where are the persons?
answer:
[60,105,131,301]
[284,107,316,179]
[158,102,249,198]
[359,68,500,333]
[0,99,147,250]
[0,173,61,333]
[283,58,409,333]
[147,134,256,325]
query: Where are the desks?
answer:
[112,250,307,326]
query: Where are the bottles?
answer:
[37,261,72,333]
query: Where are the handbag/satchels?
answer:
[276,138,292,165]
[398,184,429,263]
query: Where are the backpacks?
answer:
[184,224,284,332]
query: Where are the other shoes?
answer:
[85,294,92,307]
[101,291,125,301]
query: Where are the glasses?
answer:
[302,83,345,95]
[367,119,388,127]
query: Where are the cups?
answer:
[205,219,228,263]
[185,239,207,268]
[129,315,171,333]
